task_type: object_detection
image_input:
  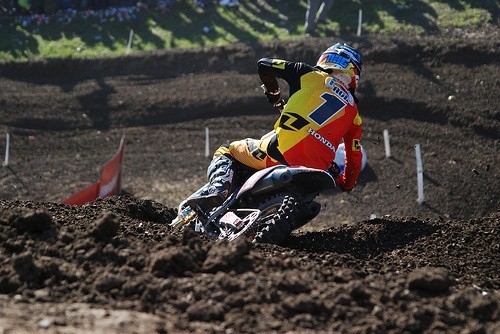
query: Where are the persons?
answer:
[167,41,366,244]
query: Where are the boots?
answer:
[181,156,234,212]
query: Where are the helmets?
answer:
[315,42,364,90]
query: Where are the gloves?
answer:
[264,88,281,104]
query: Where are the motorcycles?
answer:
[170,84,354,245]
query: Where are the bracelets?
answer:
[268,86,282,97]
